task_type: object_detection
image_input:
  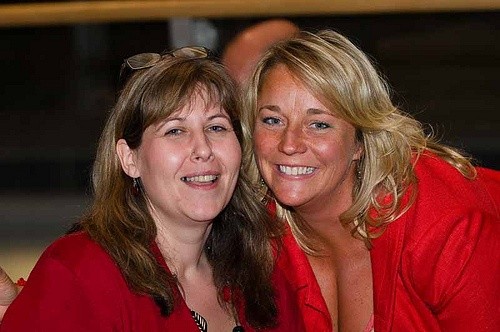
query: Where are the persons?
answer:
[201,29,500,332]
[0,44,288,332]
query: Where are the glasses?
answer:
[113,44,219,98]
